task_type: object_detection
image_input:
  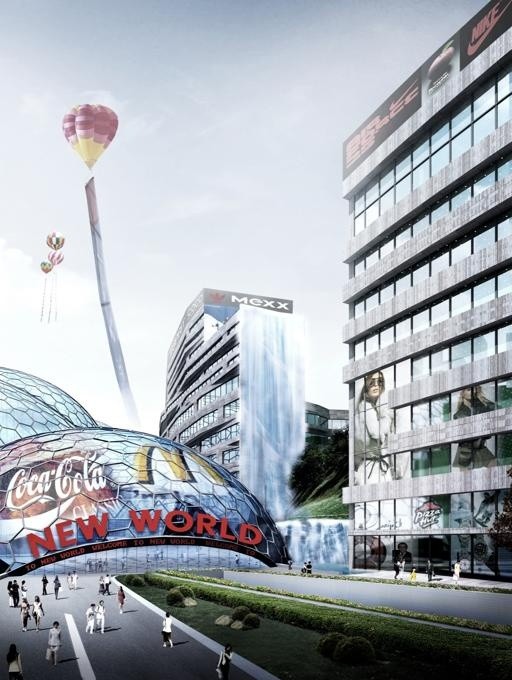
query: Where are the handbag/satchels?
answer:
[45,648,52,660]
[354,400,365,472]
[458,447,472,466]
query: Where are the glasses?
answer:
[367,377,383,387]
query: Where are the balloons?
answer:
[47,250,65,266]
[46,232,66,250]
[61,103,118,171]
[41,261,53,274]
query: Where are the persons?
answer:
[6,581,14,607]
[408,567,417,582]
[85,552,162,573]
[287,558,293,570]
[85,603,95,634]
[47,621,62,666]
[41,575,48,595]
[53,575,61,600]
[452,384,502,524]
[103,573,111,595]
[98,576,105,594]
[302,562,308,574]
[95,600,106,634]
[425,559,435,582]
[453,560,460,584]
[20,580,27,599]
[20,598,31,632]
[6,644,23,680]
[306,561,312,574]
[392,555,399,579]
[354,370,393,529]
[31,595,44,631]
[117,586,125,614]
[393,542,411,563]
[396,557,405,579]
[215,643,233,680]
[235,555,239,567]
[12,580,19,607]
[67,572,72,590]
[161,612,174,648]
[71,570,78,590]
